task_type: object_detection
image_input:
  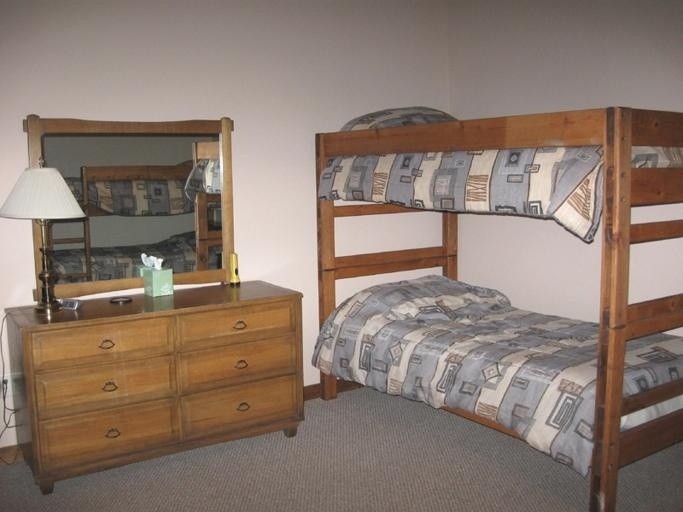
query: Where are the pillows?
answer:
[336,103,461,135]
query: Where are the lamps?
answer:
[0,157,88,314]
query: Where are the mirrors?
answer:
[25,114,237,305]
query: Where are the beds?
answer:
[44,141,226,295]
[310,106,682,511]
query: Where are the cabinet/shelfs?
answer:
[4,277,305,497]
